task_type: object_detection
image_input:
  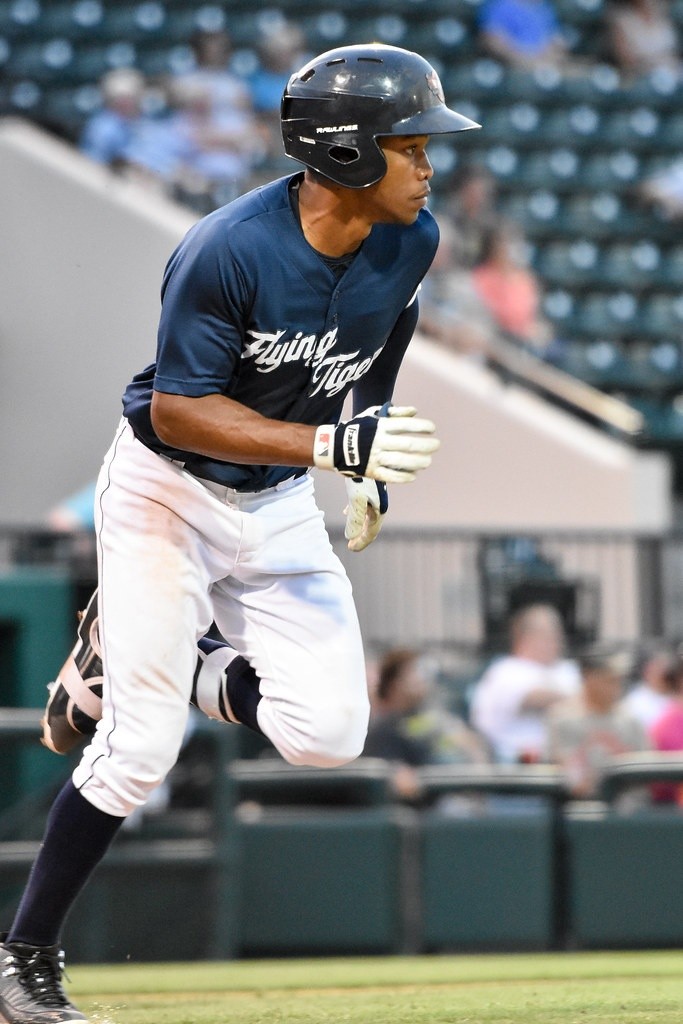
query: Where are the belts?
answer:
[133,430,308,493]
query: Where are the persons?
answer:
[78,0,683,377]
[0,42,482,1024]
[362,580,683,824]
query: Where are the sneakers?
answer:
[0,932,88,1024]
[39,587,103,755]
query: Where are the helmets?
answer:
[280,43,483,188]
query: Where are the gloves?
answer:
[343,474,388,551]
[314,401,441,484]
